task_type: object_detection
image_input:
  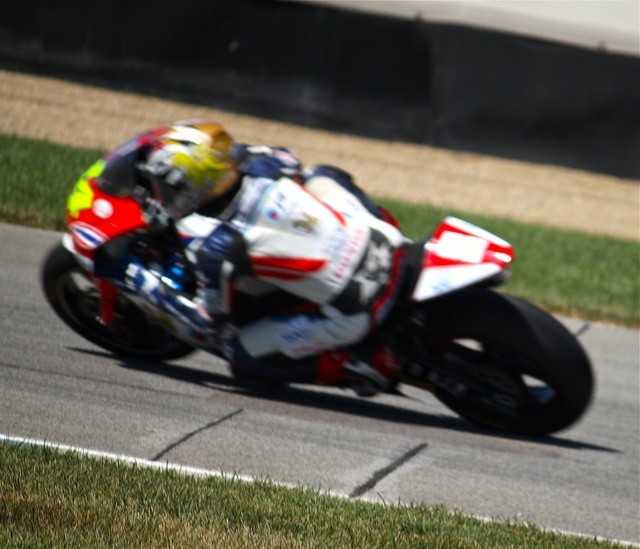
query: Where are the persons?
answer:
[124,118,409,398]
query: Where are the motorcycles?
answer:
[39,130,594,439]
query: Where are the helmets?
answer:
[143,120,239,218]
